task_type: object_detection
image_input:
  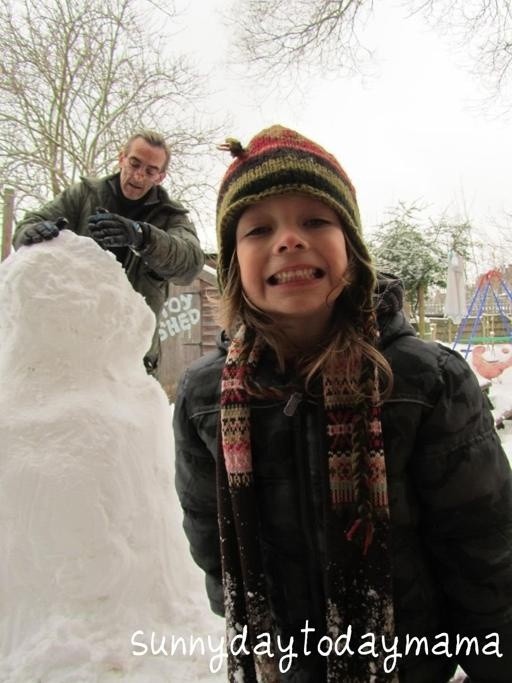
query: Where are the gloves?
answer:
[19,217,69,246]
[86,206,143,250]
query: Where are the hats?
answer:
[216,124,372,296]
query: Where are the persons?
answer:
[172,125,511,683]
[12,128,205,375]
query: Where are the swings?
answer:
[471,276,511,379]
